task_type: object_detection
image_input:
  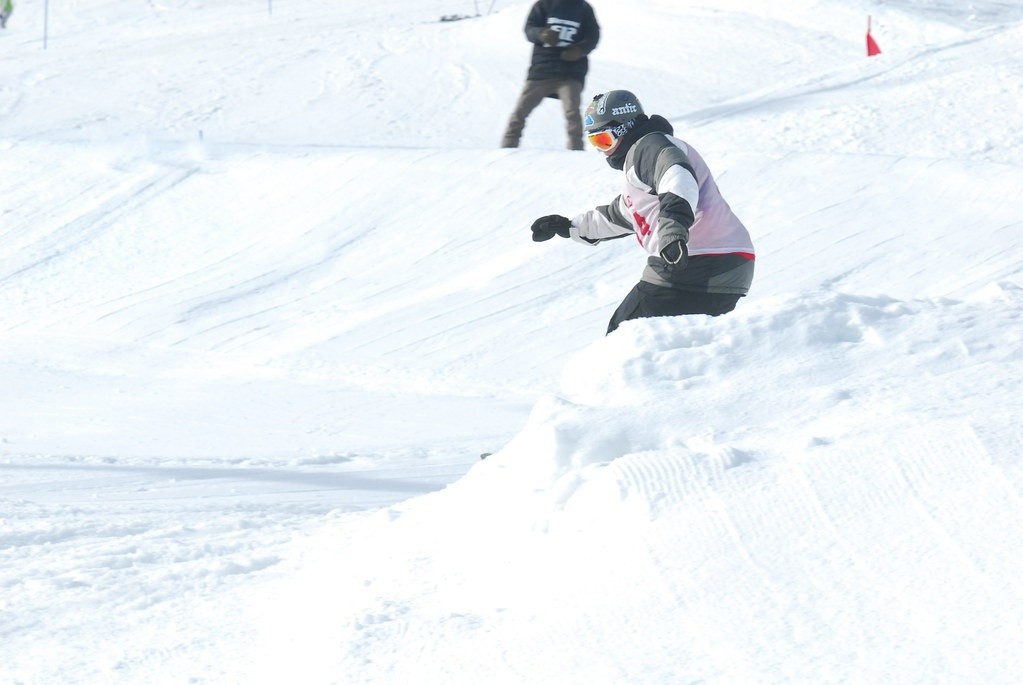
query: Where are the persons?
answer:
[532,90,755,336]
[499,0,600,152]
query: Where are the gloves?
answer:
[660,240,688,273]
[540,29,558,47]
[561,45,583,61]
[531,214,571,242]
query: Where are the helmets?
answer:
[585,90,643,131]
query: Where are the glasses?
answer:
[587,129,618,153]
